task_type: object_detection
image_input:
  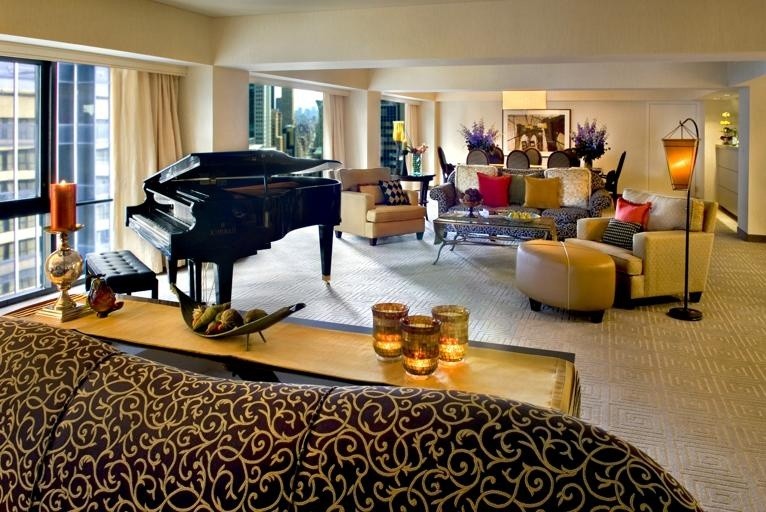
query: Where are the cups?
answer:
[372,301,469,383]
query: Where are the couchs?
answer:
[428,167,616,239]
[0,314,706,511]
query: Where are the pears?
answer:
[509,210,531,220]
[87,277,116,313]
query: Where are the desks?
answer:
[396,174,436,221]
[0,289,585,421]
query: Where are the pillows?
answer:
[454,164,499,196]
[500,171,539,205]
[476,171,511,207]
[521,174,562,209]
[598,217,641,248]
[378,178,412,207]
[356,182,386,205]
[615,196,653,232]
[543,167,593,210]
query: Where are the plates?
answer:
[494,210,542,224]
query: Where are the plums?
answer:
[462,188,482,203]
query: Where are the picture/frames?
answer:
[501,108,572,159]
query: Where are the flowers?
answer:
[404,132,429,173]
[569,116,615,163]
[455,116,501,159]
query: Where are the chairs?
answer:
[436,144,628,201]
[327,165,427,248]
[564,185,719,311]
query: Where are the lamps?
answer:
[660,116,708,321]
[393,120,405,175]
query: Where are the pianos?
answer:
[123,152,342,311]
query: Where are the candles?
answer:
[402,141,407,150]
[47,177,78,231]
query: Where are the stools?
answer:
[83,249,160,300]
[513,238,619,325]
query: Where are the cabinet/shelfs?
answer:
[715,143,738,220]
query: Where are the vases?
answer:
[412,153,422,176]
